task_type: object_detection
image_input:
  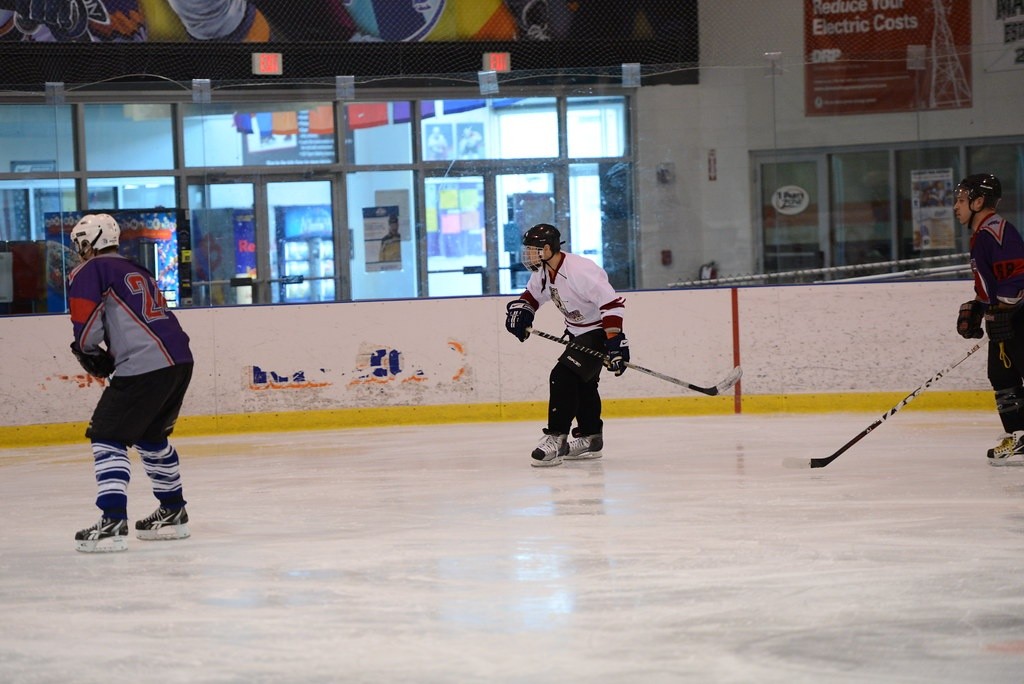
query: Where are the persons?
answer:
[377,213,401,261]
[505,224,630,467]
[68,213,194,553]
[956,172,1024,466]
[926,180,947,206]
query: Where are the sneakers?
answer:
[531,422,570,467]
[75,518,129,552]
[987,429,1024,466]
[563,421,603,459]
[134,501,189,540]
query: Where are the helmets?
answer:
[69,213,121,263]
[520,223,565,272]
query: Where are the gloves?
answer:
[602,333,630,377]
[504,298,537,343]
[955,300,985,340]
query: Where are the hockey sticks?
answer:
[524,325,743,396]
[780,335,989,468]
